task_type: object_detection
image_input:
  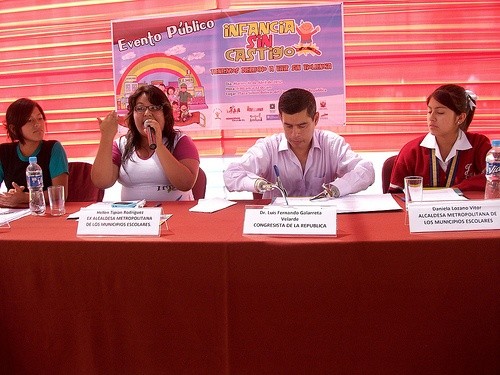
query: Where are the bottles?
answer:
[483,140,500,200]
[25,156,47,216]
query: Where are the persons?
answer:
[387,83,492,193]
[0,98,68,208]
[90,84,199,201]
[225,88,375,198]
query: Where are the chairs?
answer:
[67,162,106,202]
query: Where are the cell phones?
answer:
[112,201,135,208]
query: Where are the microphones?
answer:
[144,120,157,150]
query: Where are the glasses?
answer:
[134,104,164,112]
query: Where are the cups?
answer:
[403,175,424,212]
[47,184,67,217]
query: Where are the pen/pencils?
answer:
[274,164,279,176]
[155,203,161,207]
[395,194,405,202]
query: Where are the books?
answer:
[263,193,403,214]
[0,208,31,226]
[66,199,146,218]
[402,187,471,201]
[189,197,238,213]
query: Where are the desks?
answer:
[0,191,500,375]
